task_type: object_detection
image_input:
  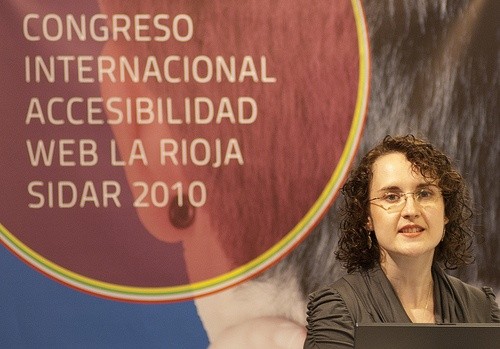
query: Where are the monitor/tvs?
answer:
[354,323,500,349]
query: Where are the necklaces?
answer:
[401,278,433,325]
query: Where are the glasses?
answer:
[365,186,444,206]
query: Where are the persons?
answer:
[299,125,500,348]
